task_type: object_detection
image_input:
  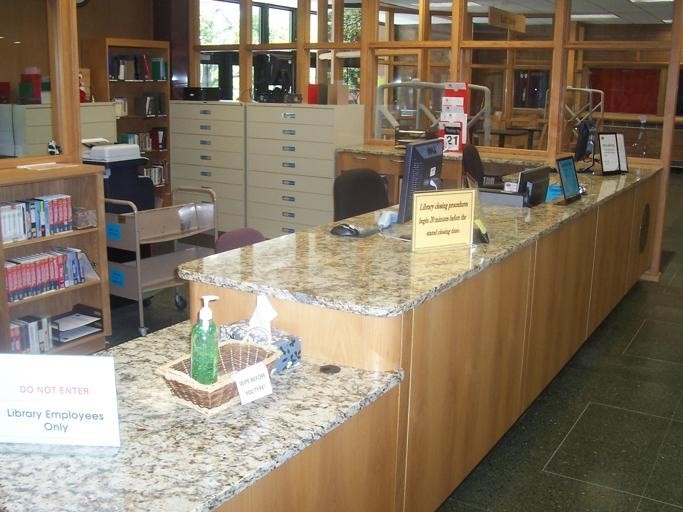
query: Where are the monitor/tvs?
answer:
[575,119,597,173]
[517,165,550,207]
[396,138,445,241]
[555,156,581,205]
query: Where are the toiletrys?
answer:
[190,294,221,385]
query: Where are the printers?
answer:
[80,137,155,310]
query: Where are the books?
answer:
[108,50,168,187]
[0,194,101,355]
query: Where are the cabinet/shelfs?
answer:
[168,101,365,239]
[0,169,110,353]
[81,37,171,206]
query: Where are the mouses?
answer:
[550,167,557,173]
[330,223,359,237]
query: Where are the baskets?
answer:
[155,338,282,414]
